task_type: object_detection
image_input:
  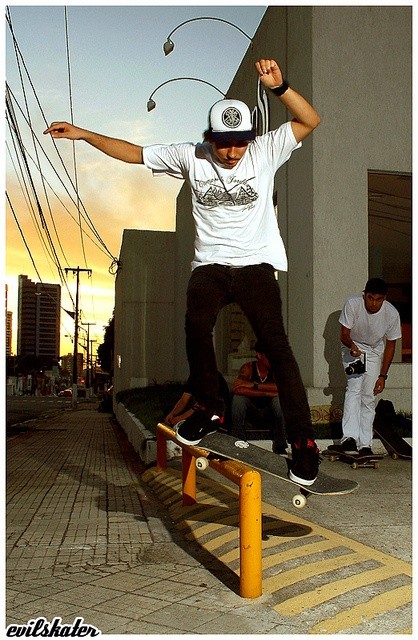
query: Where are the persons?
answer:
[41,59,322,487]
[338,279,402,456]
[164,369,230,425]
[231,343,287,455]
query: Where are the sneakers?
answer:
[289,435,319,487]
[174,400,224,446]
[359,447,373,456]
[340,437,359,456]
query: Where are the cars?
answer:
[58,389,72,396]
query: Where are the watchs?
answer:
[378,374,388,380]
[253,380,259,389]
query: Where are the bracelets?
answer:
[270,79,289,96]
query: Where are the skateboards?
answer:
[176,419,360,509]
[373,416,412,460]
[321,449,385,470]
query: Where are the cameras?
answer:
[345,350,367,376]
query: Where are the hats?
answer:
[207,98,255,144]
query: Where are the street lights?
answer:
[64,334,90,388]
[35,291,79,405]
